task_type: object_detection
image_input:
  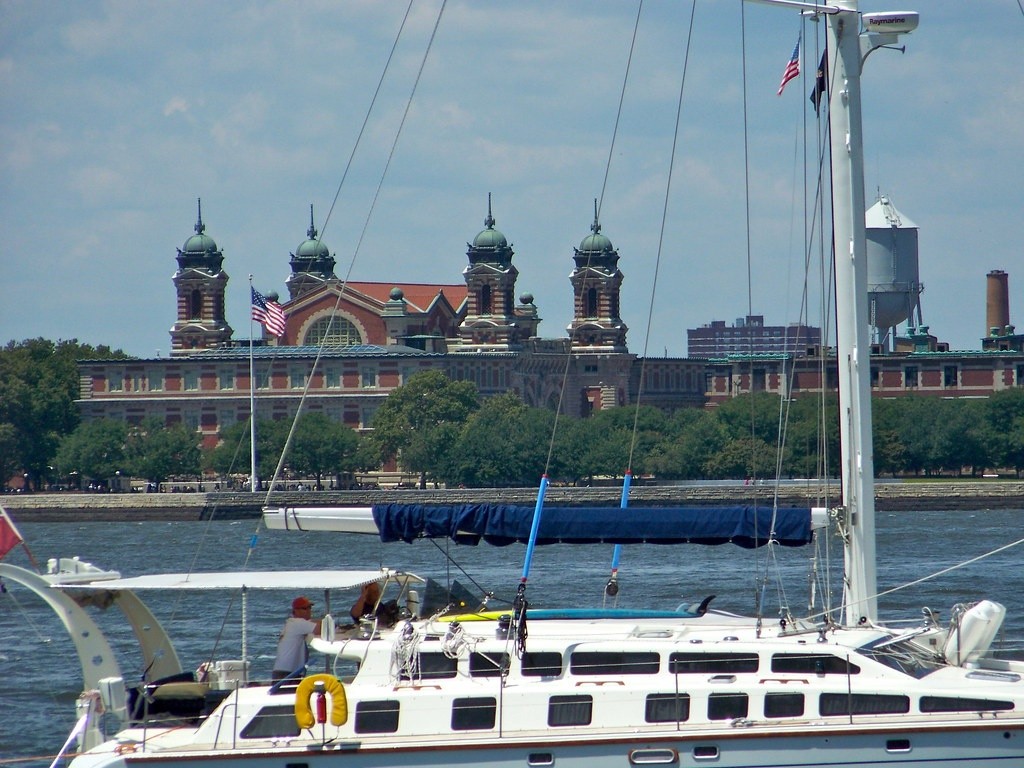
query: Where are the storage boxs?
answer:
[202,660,253,690]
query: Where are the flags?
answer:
[0,512,20,563]
[810,50,826,118]
[250,286,285,338]
[778,35,800,96]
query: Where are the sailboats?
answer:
[0,2,1023,768]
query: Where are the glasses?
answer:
[299,606,311,610]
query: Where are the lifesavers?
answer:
[294,674,348,729]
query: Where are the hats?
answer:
[292,598,314,609]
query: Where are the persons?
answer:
[760,478,764,485]
[0,477,382,494]
[459,485,464,489]
[751,477,755,485]
[350,582,385,670]
[744,477,749,485]
[272,598,324,686]
